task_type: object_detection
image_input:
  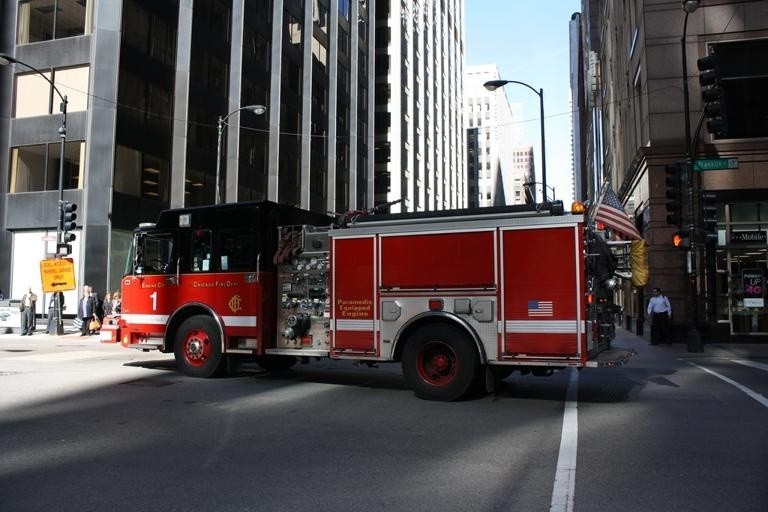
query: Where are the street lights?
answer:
[216,105,268,210]
[481,78,549,203]
[2,55,68,338]
[681,0,710,352]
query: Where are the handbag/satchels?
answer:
[73,318,83,328]
[89,321,100,331]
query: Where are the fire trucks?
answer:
[97,200,639,404]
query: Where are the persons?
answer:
[647,286,672,348]
[0,280,120,338]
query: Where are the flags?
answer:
[594,186,643,243]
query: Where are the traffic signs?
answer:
[40,257,77,292]
[694,157,738,171]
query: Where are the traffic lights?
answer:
[665,163,683,226]
[697,48,729,137]
[702,194,718,245]
[63,203,77,244]
[673,232,690,250]
